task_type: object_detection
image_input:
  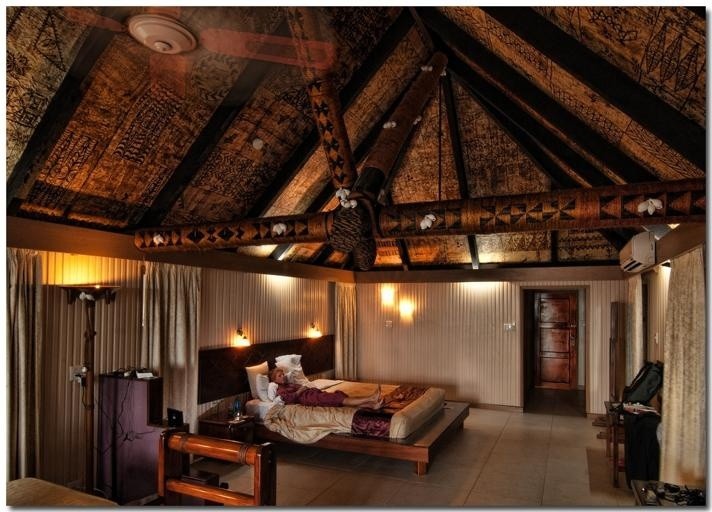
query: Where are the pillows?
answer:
[245,353,310,402]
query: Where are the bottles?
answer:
[234,394,241,416]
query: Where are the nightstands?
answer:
[200,412,255,464]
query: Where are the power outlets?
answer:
[69,365,82,383]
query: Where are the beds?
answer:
[245,378,471,475]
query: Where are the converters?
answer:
[79,377,88,389]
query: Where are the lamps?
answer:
[307,321,321,339]
[234,327,250,349]
[59,284,121,497]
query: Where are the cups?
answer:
[233,412,242,421]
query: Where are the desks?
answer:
[603,400,661,487]
[630,479,707,507]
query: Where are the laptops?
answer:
[167,407,184,429]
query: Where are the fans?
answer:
[55,7,337,102]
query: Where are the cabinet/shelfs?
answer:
[95,371,191,504]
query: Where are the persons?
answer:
[269,367,385,410]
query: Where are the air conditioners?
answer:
[618,231,656,274]
